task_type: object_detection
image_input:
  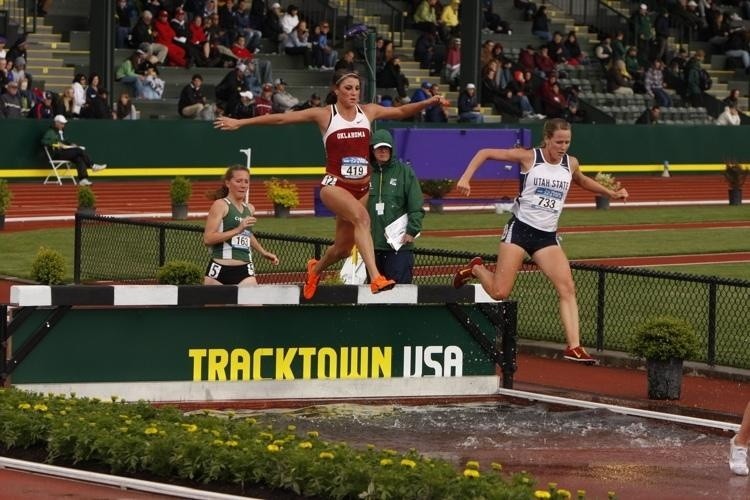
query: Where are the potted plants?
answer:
[628,317,706,401]
[264,178,301,218]
[420,178,456,212]
[0,179,14,229]
[595,174,622,208]
[77,187,96,223]
[720,162,750,205]
[170,177,191,219]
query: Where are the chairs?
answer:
[43,145,77,186]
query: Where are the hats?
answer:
[53,114,68,124]
[158,7,184,18]
[235,63,286,100]
[372,142,393,150]
[421,80,432,89]
[14,56,26,66]
[466,82,475,89]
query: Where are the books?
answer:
[384,213,421,251]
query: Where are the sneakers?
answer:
[369,275,397,295]
[560,345,597,365]
[728,434,750,475]
[80,178,93,186]
[92,163,107,172]
[302,258,323,301]
[451,256,487,289]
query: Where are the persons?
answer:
[0,2,750,124]
[214,67,451,300]
[728,400,750,477]
[40,115,107,188]
[452,118,630,362]
[367,126,427,283]
[199,167,279,307]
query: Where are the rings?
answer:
[247,221,249,224]
[221,122,224,127]
